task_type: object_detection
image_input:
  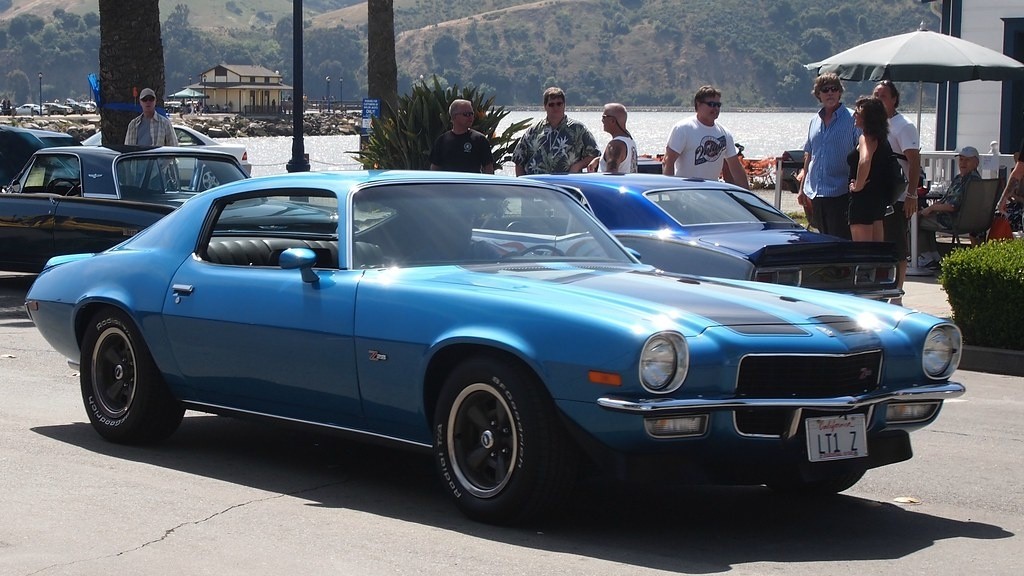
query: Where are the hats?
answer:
[954,146,980,163]
[140,88,156,100]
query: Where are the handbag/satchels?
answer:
[880,141,910,206]
[986,210,1015,243]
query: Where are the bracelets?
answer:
[906,196,917,200]
[907,192,918,197]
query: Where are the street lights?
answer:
[326,75,331,111]
[203,74,207,111]
[38,71,43,116]
[339,76,343,111]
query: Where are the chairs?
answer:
[922,179,1002,269]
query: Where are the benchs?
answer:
[208,240,386,269]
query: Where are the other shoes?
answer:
[906,253,942,270]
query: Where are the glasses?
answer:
[820,85,839,93]
[602,115,615,122]
[876,80,891,86]
[141,96,155,102]
[455,112,474,116]
[853,109,865,121]
[702,101,722,108]
[549,102,563,107]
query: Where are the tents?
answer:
[168,87,210,113]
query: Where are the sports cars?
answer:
[23,170,965,528]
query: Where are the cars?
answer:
[80,121,252,192]
[470,171,906,308]
[0,146,336,276]
[17,100,97,116]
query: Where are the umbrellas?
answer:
[804,19,1024,151]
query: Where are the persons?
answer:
[798,71,1024,309]
[288,94,337,112]
[587,103,638,174]
[122,88,179,190]
[660,84,749,201]
[430,100,498,216]
[2,99,11,116]
[512,87,600,218]
[418,203,502,262]
[179,101,233,115]
